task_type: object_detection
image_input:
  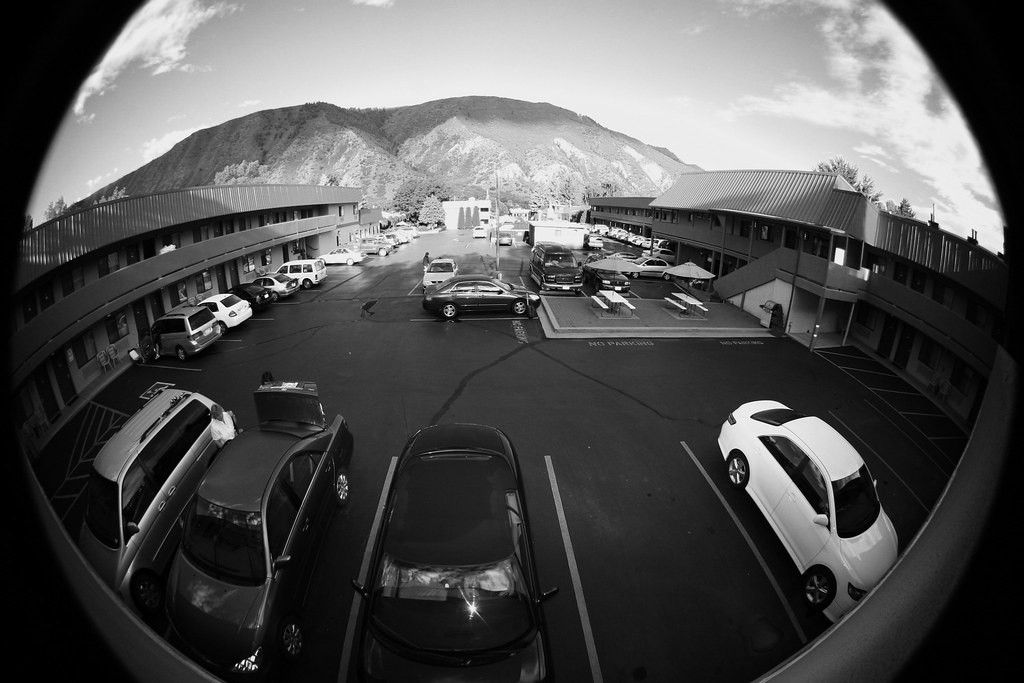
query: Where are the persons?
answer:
[423,252,430,270]
[210,404,237,449]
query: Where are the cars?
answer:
[473,226,487,239]
[196,293,253,337]
[498,232,513,246]
[350,424,559,682]
[592,224,672,249]
[607,251,637,260]
[423,273,543,319]
[421,258,463,292]
[356,223,421,259]
[154,380,358,683]
[622,256,676,282]
[224,283,275,309]
[642,247,676,263]
[718,400,899,623]
[317,247,366,266]
[584,254,631,295]
[251,273,301,298]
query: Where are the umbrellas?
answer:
[663,262,716,307]
[586,255,644,314]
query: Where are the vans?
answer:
[584,234,604,250]
[272,259,328,291]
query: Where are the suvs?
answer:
[79,387,240,623]
[528,241,583,296]
[144,306,222,361]
